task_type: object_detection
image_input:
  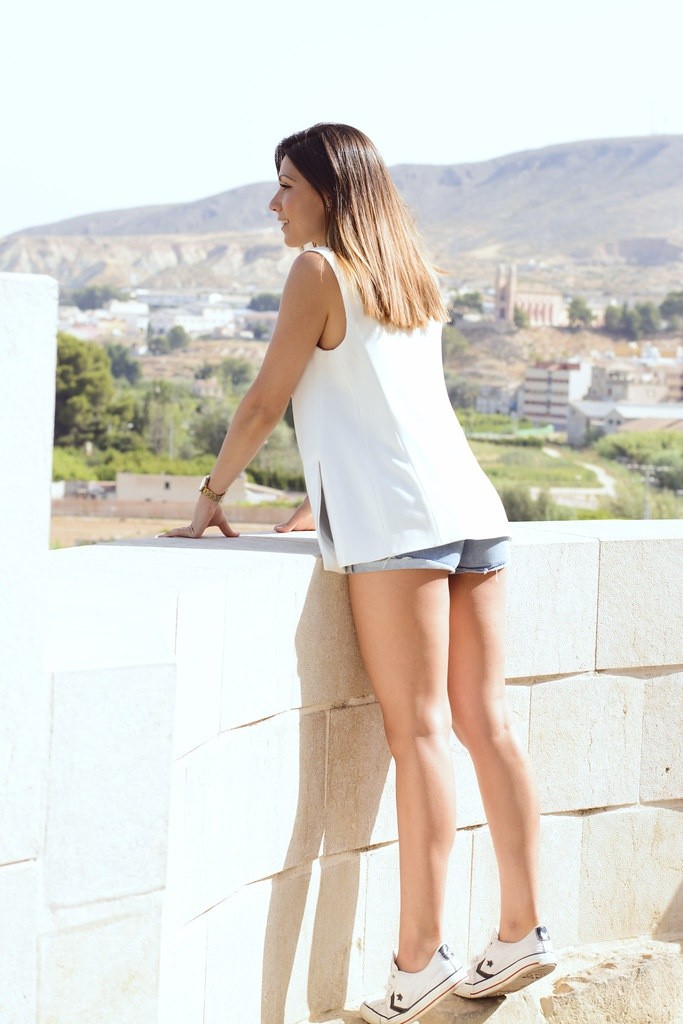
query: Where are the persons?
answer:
[152,119,563,1024]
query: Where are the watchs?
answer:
[198,475,229,503]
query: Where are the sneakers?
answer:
[358,946,468,1024]
[454,926,559,997]
